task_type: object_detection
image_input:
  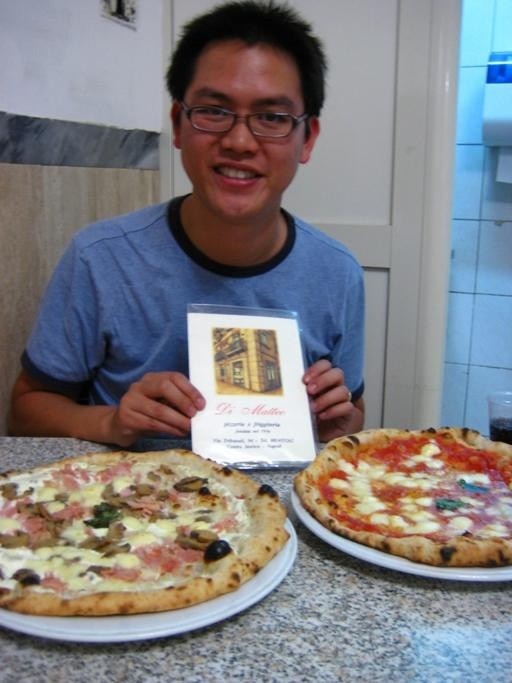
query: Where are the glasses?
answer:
[178,98,309,138]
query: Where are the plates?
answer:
[291,485,512,582]
[1,517,297,644]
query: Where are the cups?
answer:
[486,392,512,444]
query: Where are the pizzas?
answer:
[295,425,512,567]
[0,449,290,618]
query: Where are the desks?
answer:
[0,433,512,682]
